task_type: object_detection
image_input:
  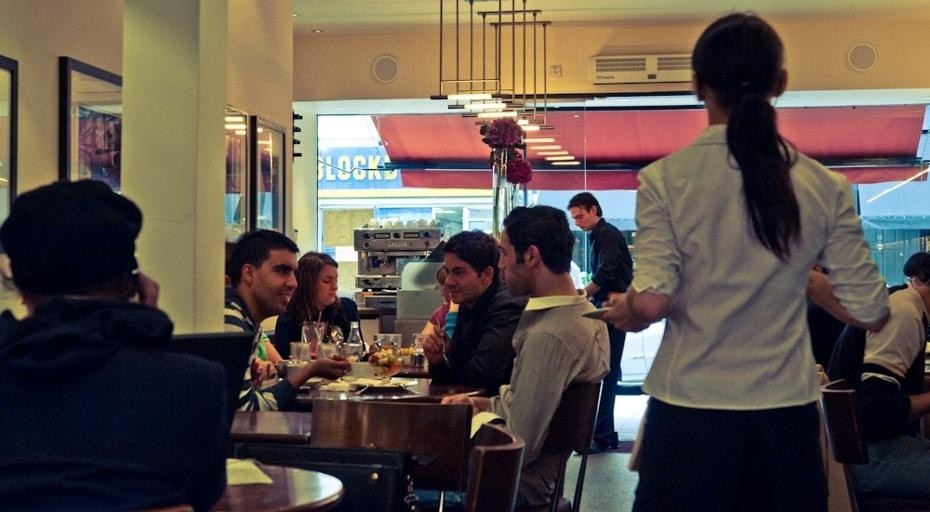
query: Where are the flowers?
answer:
[480,117,533,201]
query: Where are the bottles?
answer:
[346,321,363,355]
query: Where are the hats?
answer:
[1,178,144,297]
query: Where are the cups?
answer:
[410,351,425,368]
[290,321,336,365]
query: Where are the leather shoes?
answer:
[575,435,609,453]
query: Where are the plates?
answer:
[582,308,611,320]
[349,376,420,389]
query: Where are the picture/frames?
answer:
[55,54,123,196]
[223,102,287,256]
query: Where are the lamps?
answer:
[428,0,556,136]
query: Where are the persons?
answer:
[441,207,610,512]
[225,229,351,413]
[808,251,930,512]
[567,192,632,451]
[589,10,893,510]
[422,229,526,397]
[275,253,368,361]
[0,175,232,510]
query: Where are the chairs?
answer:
[467,376,605,511]
[819,379,870,512]
[232,409,311,452]
[308,398,473,512]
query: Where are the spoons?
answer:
[437,319,452,369]
